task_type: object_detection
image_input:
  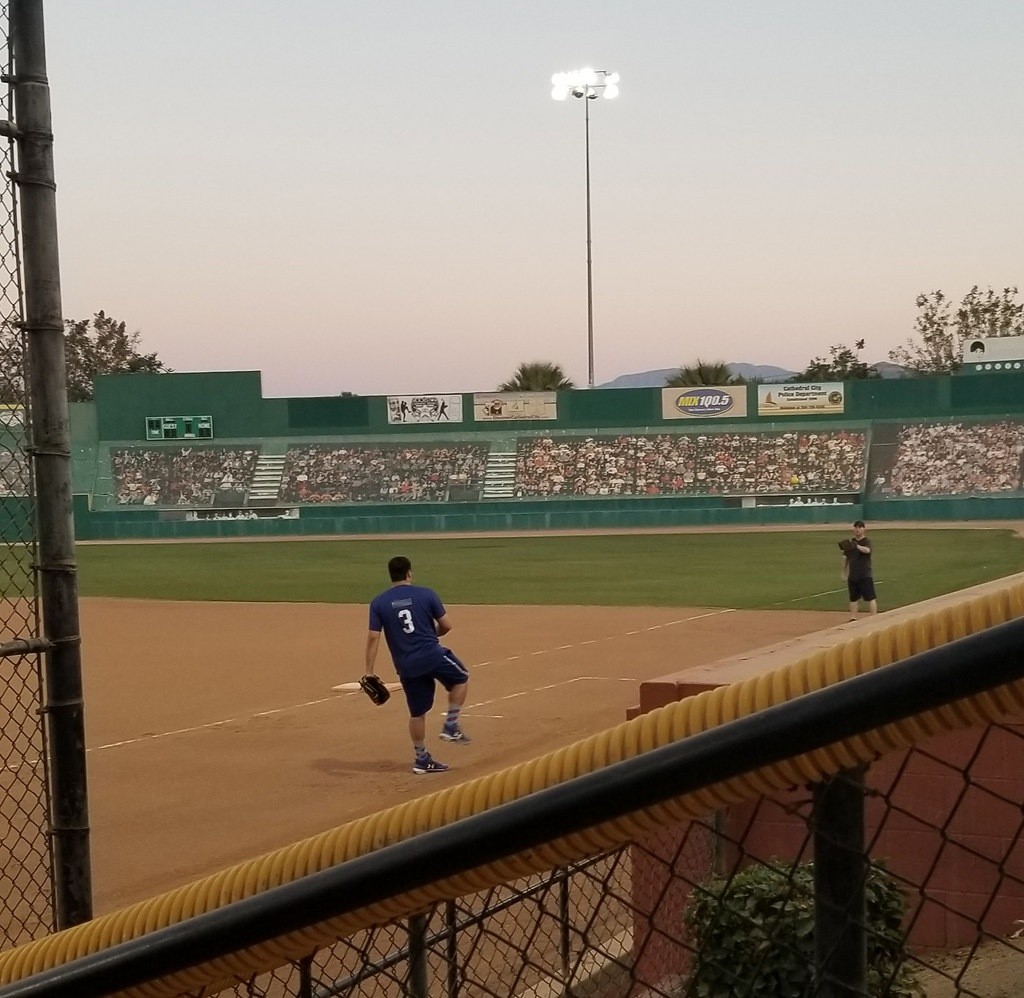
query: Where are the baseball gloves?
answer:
[838,539,857,552]
[357,674,391,705]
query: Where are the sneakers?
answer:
[847,618,856,623]
[413,752,449,774]
[438,723,473,745]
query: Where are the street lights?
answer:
[547,67,620,389]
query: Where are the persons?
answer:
[111,418,1024,511]
[367,555,471,774]
[840,521,877,624]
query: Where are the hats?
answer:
[853,521,865,528]
